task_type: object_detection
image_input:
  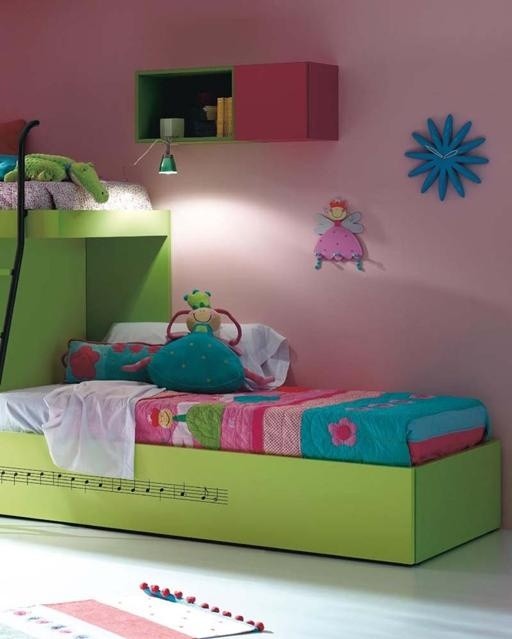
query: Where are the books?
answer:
[215,97,223,138]
[224,96,234,138]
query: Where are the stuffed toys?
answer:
[122,288,275,394]
[3,152,110,204]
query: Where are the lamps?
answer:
[133,139,176,174]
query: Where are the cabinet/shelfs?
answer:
[136,63,339,146]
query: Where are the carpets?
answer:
[1,583,264,637]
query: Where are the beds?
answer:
[1,384,504,564]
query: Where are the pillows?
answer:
[61,338,163,385]
